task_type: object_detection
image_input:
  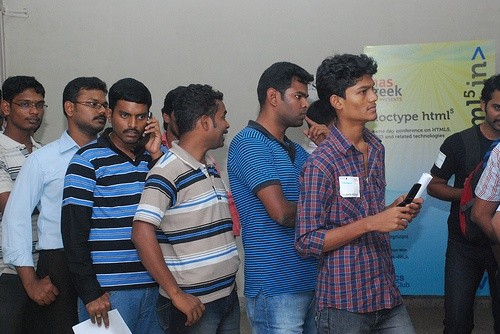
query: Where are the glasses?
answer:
[5,99,48,110]
[73,101,110,109]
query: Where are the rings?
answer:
[97,315,101,317]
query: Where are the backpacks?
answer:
[460,138,500,246]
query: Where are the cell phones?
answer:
[131,111,152,152]
[398,183,421,207]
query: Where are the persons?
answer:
[304,99,337,155]
[0,76,47,334]
[425,74,500,334]
[2,76,109,334]
[470,141,500,265]
[160,85,241,239]
[60,77,165,334]
[227,61,331,334]
[130,84,240,333]
[295,53,423,334]
